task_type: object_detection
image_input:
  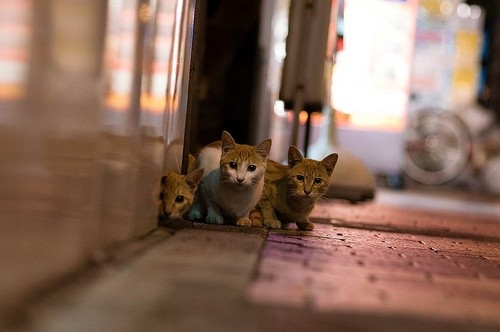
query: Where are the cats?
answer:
[189,131,272,228]
[259,145,339,231]
[158,167,206,224]
